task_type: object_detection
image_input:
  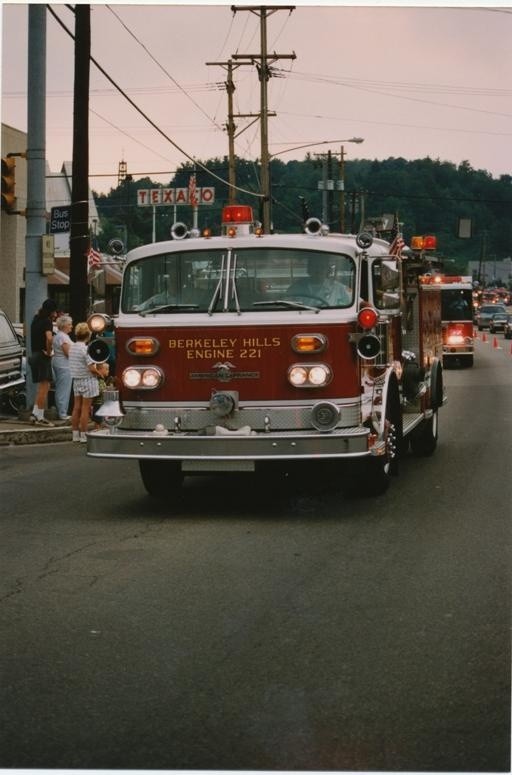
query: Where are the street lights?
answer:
[260,137,364,232]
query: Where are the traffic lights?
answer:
[2,156,14,213]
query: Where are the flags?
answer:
[388,215,405,261]
[188,164,198,209]
[88,224,101,270]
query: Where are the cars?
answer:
[477,303,511,339]
[0,310,26,396]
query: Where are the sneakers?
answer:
[73,436,87,443]
[29,414,55,427]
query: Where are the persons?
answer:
[50,308,67,388]
[130,268,199,312]
[29,298,56,428]
[50,315,75,421]
[90,361,111,432]
[286,252,351,307]
[68,321,105,444]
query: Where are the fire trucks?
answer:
[86,206,445,501]
[419,274,475,368]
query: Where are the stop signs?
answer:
[50,204,71,234]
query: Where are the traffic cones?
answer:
[473,330,477,337]
[494,337,499,349]
[483,333,487,342]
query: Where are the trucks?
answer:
[479,288,510,304]
